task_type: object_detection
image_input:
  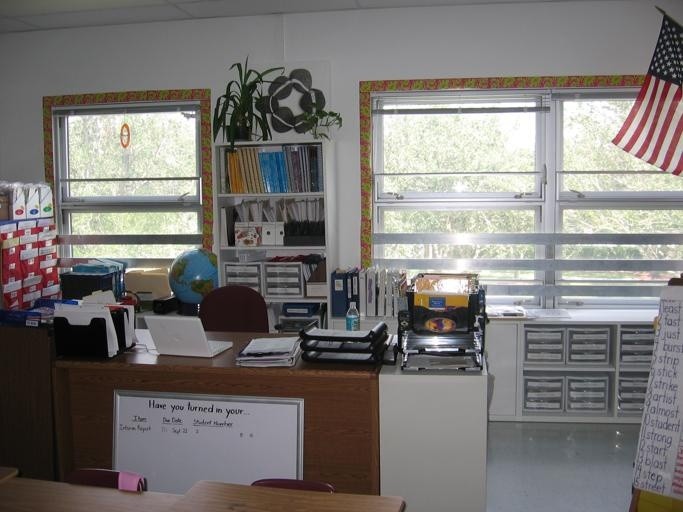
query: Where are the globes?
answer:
[169,249,218,315]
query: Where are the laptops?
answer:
[144,315,233,358]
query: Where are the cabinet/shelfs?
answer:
[516,311,656,423]
[213,138,339,331]
[357,307,520,424]
[378,336,487,511]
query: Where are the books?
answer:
[226,144,323,194]
[220,198,324,248]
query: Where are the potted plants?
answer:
[210,55,285,146]
[302,109,342,140]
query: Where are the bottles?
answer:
[345,301,360,330]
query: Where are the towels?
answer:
[117,469,146,491]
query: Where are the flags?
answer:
[610,15,683,177]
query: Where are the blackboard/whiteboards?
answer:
[112,389,304,493]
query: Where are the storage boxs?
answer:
[56,264,127,304]
[262,259,306,298]
[0,216,59,313]
[404,271,482,336]
[221,258,263,298]
[522,324,657,415]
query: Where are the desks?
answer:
[0,474,185,511]
[0,312,57,483]
[52,332,377,511]
[0,465,20,486]
[163,478,406,511]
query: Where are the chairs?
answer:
[249,476,337,494]
[65,465,147,492]
[196,285,270,333]
